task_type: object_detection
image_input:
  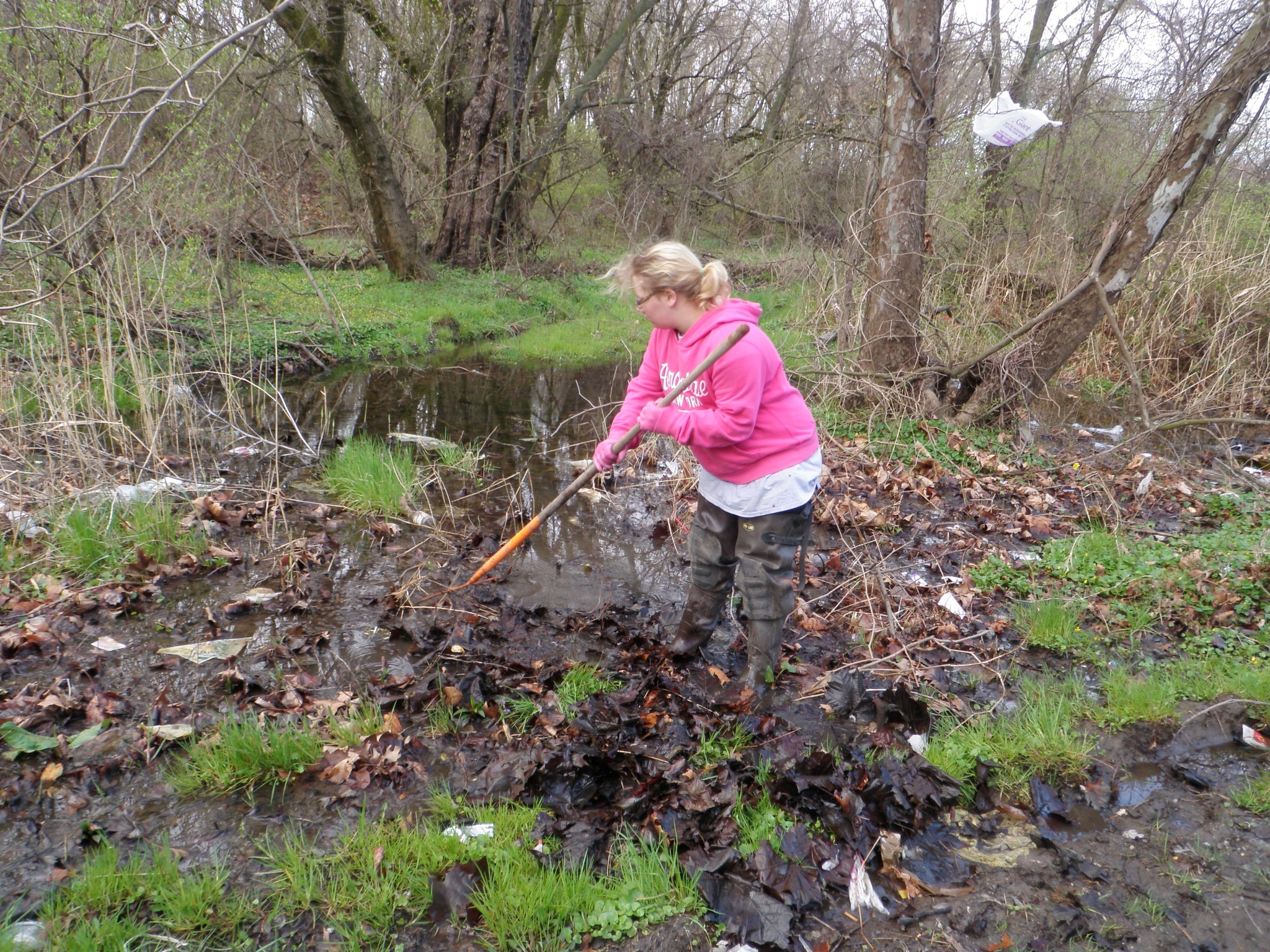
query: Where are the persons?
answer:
[593,240,822,708]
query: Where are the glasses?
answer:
[635,288,660,308]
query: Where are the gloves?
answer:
[591,439,628,472]
[639,398,668,431]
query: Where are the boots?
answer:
[647,589,734,669]
[716,617,783,708]
